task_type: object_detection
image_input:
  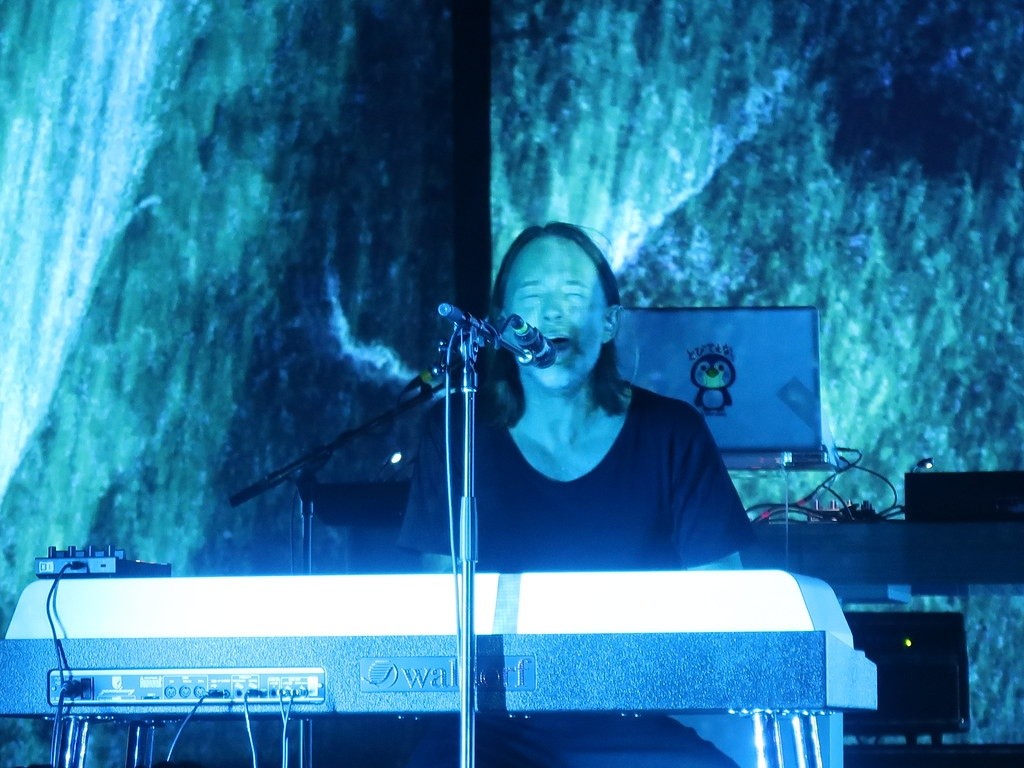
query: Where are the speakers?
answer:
[843,609,970,746]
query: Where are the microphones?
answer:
[402,343,469,392]
[510,315,557,368]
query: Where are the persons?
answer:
[394,224,754,768]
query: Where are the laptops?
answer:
[612,305,849,474]
